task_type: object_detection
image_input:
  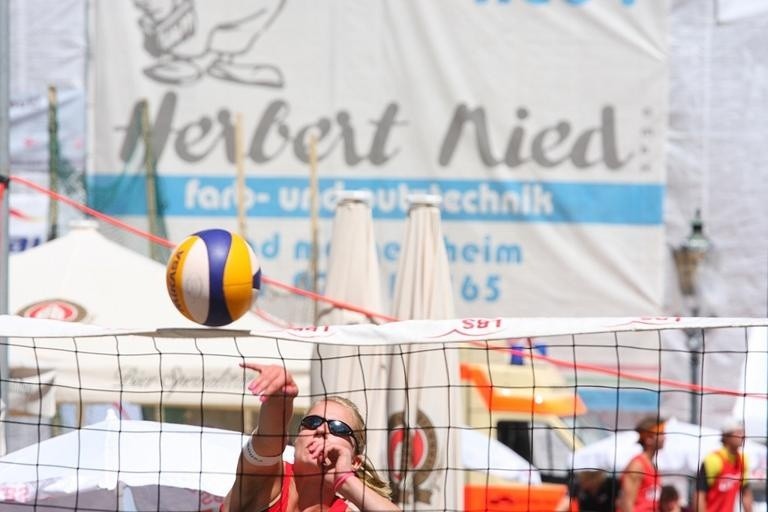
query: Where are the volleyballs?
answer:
[166,228,263,327]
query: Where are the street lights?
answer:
[671,210,713,425]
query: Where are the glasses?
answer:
[299,415,359,453]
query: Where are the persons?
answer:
[218,361,403,512]
[554,415,683,512]
[692,418,753,511]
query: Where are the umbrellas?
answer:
[383,194,463,511]
[308,188,389,498]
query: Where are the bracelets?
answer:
[332,468,355,495]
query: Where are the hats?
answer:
[721,416,745,435]
[570,450,612,473]
